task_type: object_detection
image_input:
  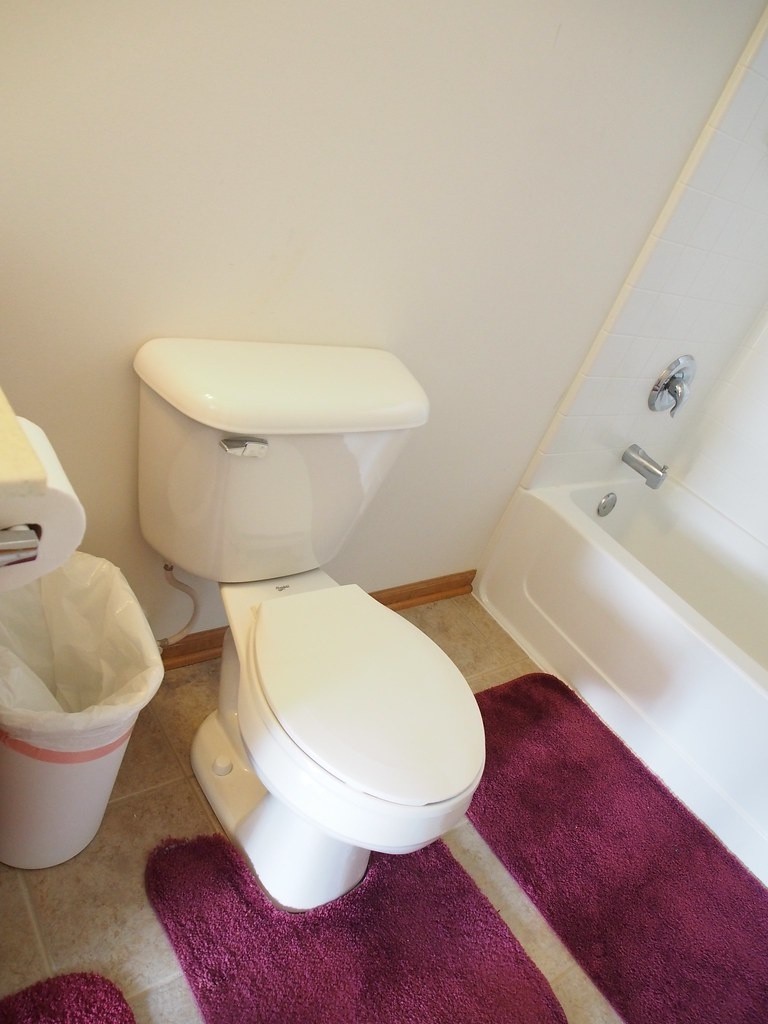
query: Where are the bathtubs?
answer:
[471,471,768,888]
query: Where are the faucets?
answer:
[621,443,669,490]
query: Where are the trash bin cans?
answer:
[0,552,167,871]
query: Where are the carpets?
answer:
[466,672,768,1024]
[143,833,568,1024]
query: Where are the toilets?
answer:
[130,336,490,914]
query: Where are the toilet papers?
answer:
[0,415,89,594]
